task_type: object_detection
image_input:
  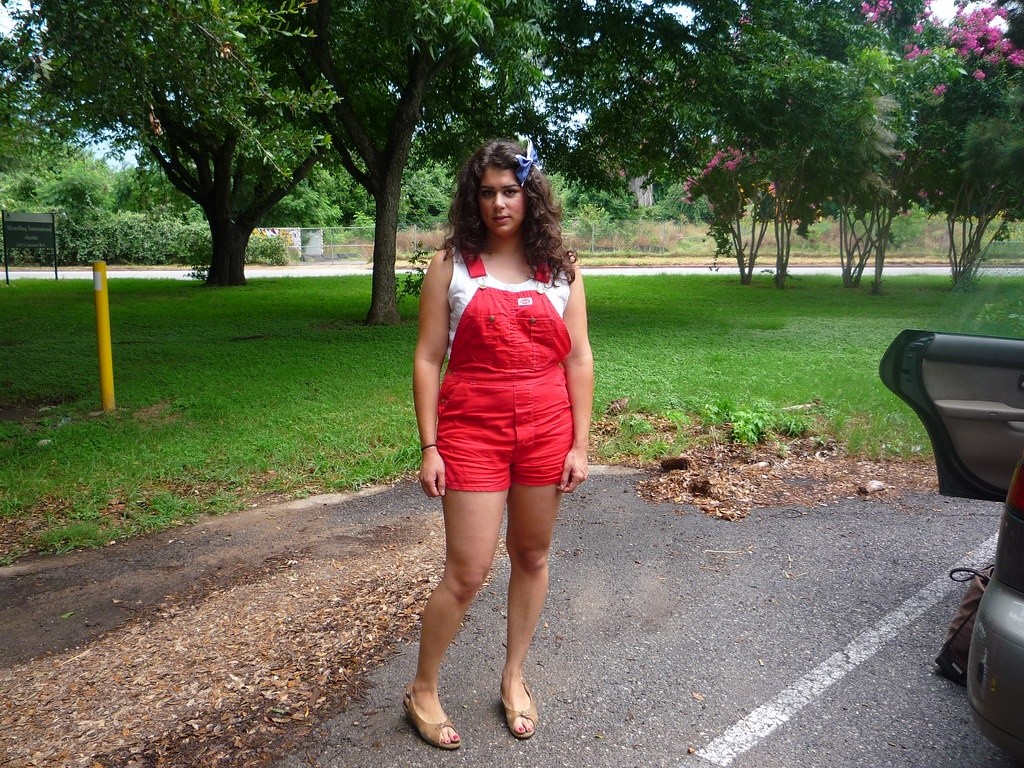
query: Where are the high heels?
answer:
[500,680,538,739]
[402,685,464,750]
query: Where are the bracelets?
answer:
[421,444,437,451]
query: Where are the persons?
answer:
[401,135,593,751]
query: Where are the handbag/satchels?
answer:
[937,563,998,684]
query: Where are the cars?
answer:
[875,327,1022,763]
